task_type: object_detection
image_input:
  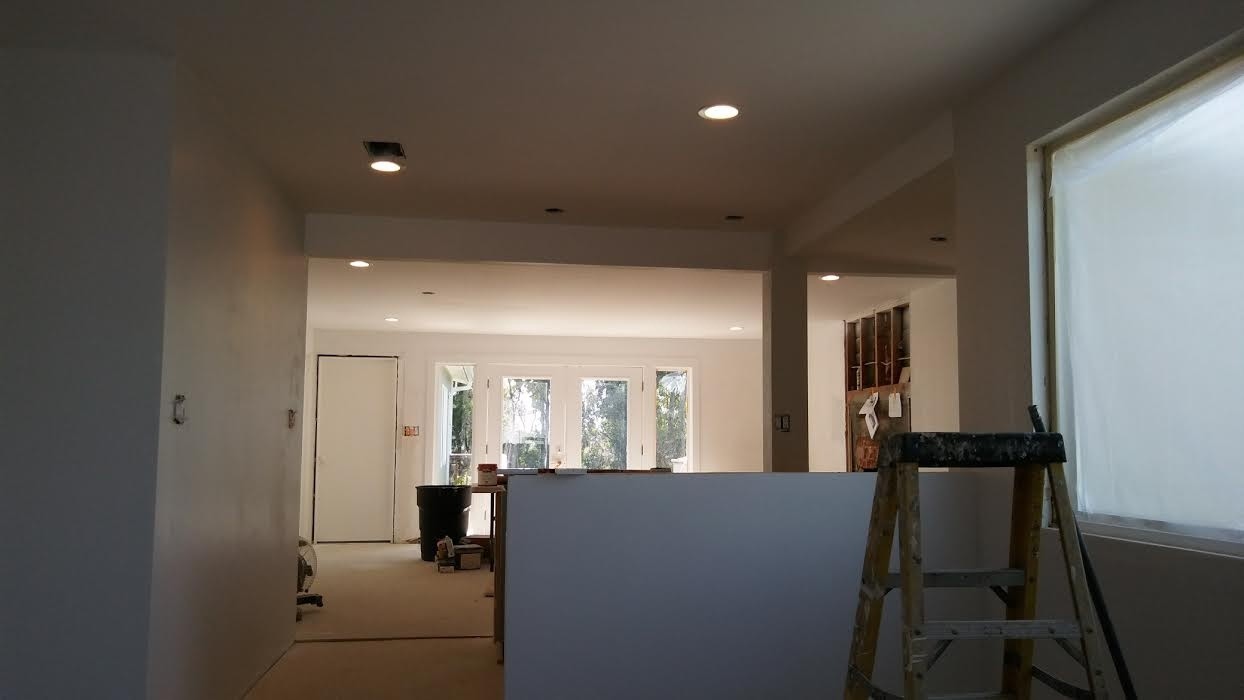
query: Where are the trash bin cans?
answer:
[416,485,471,561]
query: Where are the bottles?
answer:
[445,536,455,557]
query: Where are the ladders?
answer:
[845,432,1116,700]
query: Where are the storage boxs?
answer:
[455,544,483,569]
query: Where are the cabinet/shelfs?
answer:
[845,299,911,471]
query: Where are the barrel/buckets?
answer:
[478,464,497,486]
[416,485,472,561]
